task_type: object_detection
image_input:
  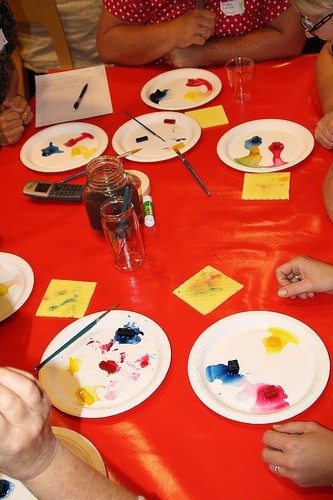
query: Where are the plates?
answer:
[0,252,34,323]
[216,117,316,174]
[20,121,109,172]
[140,67,222,111]
[187,310,330,424]
[36,309,172,417]
[112,111,202,161]
[50,425,107,479]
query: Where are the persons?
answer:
[262,256,333,491]
[0,366,141,500]
[97,0,333,223]
[0,0,34,145]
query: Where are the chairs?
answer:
[9,0,73,70]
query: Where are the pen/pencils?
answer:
[74,83,88,112]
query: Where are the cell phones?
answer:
[23,181,83,200]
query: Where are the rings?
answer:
[200,27,205,37]
[275,466,280,475]
[294,275,300,281]
[24,112,29,118]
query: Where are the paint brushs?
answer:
[59,148,144,184]
[122,109,165,143]
[173,146,212,197]
[32,304,119,372]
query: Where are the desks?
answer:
[0,54,333,500]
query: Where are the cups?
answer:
[100,198,146,272]
[226,57,255,102]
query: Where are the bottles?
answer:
[81,154,143,232]
